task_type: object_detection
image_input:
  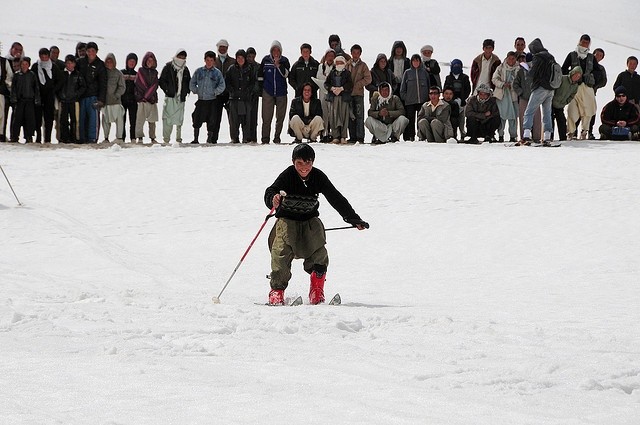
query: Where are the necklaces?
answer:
[295,175,310,187]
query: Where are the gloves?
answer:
[350,219,365,229]
[92,101,103,108]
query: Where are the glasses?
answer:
[616,94,626,97]
[429,92,438,95]
[380,88,389,92]
[14,47,23,52]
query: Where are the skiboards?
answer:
[254,293,341,306]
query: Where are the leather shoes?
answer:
[320,136,328,142]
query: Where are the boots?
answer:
[309,272,326,304]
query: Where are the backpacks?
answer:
[536,53,562,88]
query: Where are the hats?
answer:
[452,59,462,66]
[334,56,346,65]
[476,84,491,93]
[420,45,433,53]
[292,144,315,159]
[39,48,49,57]
[483,39,494,50]
[615,86,626,93]
[216,40,229,50]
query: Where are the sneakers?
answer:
[534,139,541,143]
[293,138,302,143]
[269,290,284,304]
[483,137,491,143]
[632,132,640,139]
[499,136,503,141]
[375,140,386,144]
[261,141,270,144]
[99,140,109,145]
[112,138,123,144]
[601,133,607,139]
[467,138,479,144]
[544,140,551,146]
[341,138,346,143]
[273,138,280,143]
[581,133,586,140]
[589,134,595,139]
[391,136,399,142]
[567,134,573,140]
[510,138,515,142]
[330,139,339,143]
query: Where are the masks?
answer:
[217,50,228,58]
[336,65,346,72]
[452,67,462,75]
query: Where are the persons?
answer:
[211,39,239,143]
[519,38,556,146]
[102,52,127,144]
[264,143,369,305]
[613,56,640,106]
[158,48,191,146]
[365,81,409,144]
[120,53,139,143]
[417,86,454,142]
[471,39,501,96]
[55,54,87,144]
[572,48,607,141]
[365,53,399,144]
[346,44,372,143]
[5,42,27,141]
[189,51,226,143]
[324,55,354,144]
[599,86,640,141]
[316,49,336,143]
[562,34,597,140]
[443,85,459,143]
[245,47,261,143]
[224,49,252,143]
[492,50,525,142]
[258,40,290,144]
[328,34,351,61]
[76,42,107,143]
[420,45,442,101]
[134,51,159,144]
[31,47,66,144]
[465,84,500,144]
[514,37,527,57]
[289,82,324,143]
[0,53,15,142]
[387,41,411,142]
[516,53,526,64]
[9,59,40,144]
[550,66,583,139]
[49,46,66,143]
[287,43,320,100]
[443,59,471,142]
[75,43,87,143]
[399,54,431,142]
[513,52,541,143]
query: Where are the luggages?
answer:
[515,140,531,146]
